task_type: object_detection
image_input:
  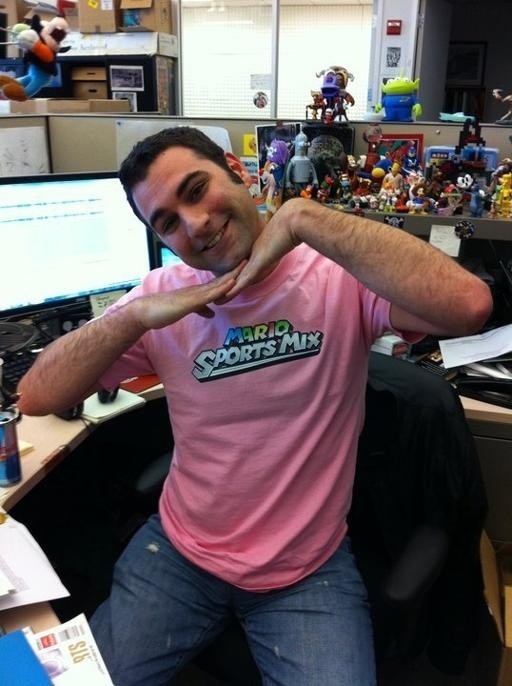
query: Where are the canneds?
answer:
[0,411,21,487]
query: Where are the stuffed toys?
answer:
[0,13,71,101]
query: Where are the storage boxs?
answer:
[1,0,177,115]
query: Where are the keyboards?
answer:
[0,340,54,404]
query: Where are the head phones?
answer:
[55,387,119,420]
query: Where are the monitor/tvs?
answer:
[156,241,184,268]
[0,170,163,322]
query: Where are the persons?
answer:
[260,64,512,218]
